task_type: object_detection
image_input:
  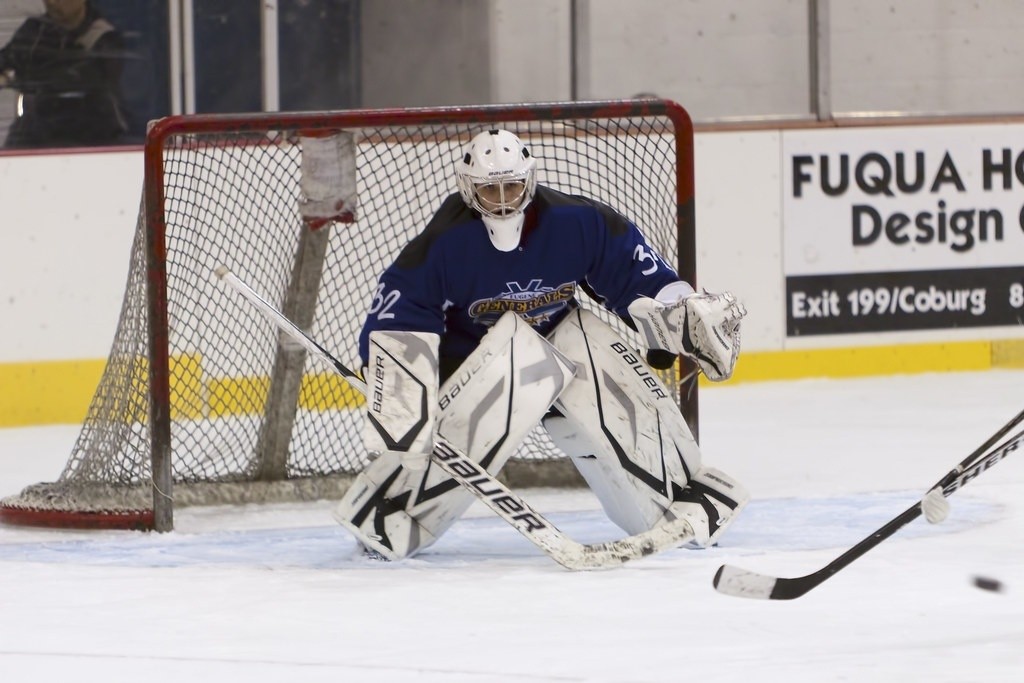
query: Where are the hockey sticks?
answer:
[713,409,1023,601]
[214,263,696,574]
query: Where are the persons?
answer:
[327,126,740,563]
[0,1,353,146]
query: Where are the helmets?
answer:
[455,130,536,252]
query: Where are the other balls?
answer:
[973,575,1001,592]
